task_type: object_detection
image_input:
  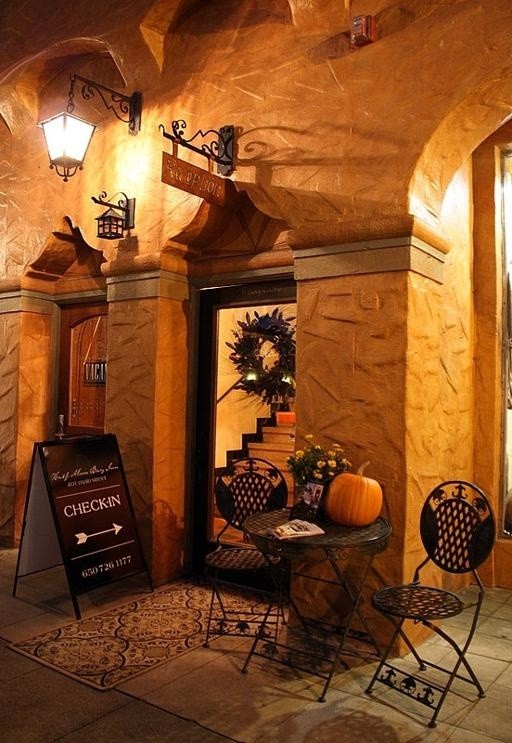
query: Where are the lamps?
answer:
[33,68,144,184]
[91,186,136,241]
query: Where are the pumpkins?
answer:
[325,460,384,527]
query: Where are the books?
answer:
[267,519,325,540]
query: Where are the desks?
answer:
[238,506,394,704]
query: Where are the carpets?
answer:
[8,578,288,695]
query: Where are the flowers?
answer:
[287,428,355,487]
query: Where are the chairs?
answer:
[363,478,497,730]
[193,455,288,654]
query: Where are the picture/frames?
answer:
[301,478,327,517]
